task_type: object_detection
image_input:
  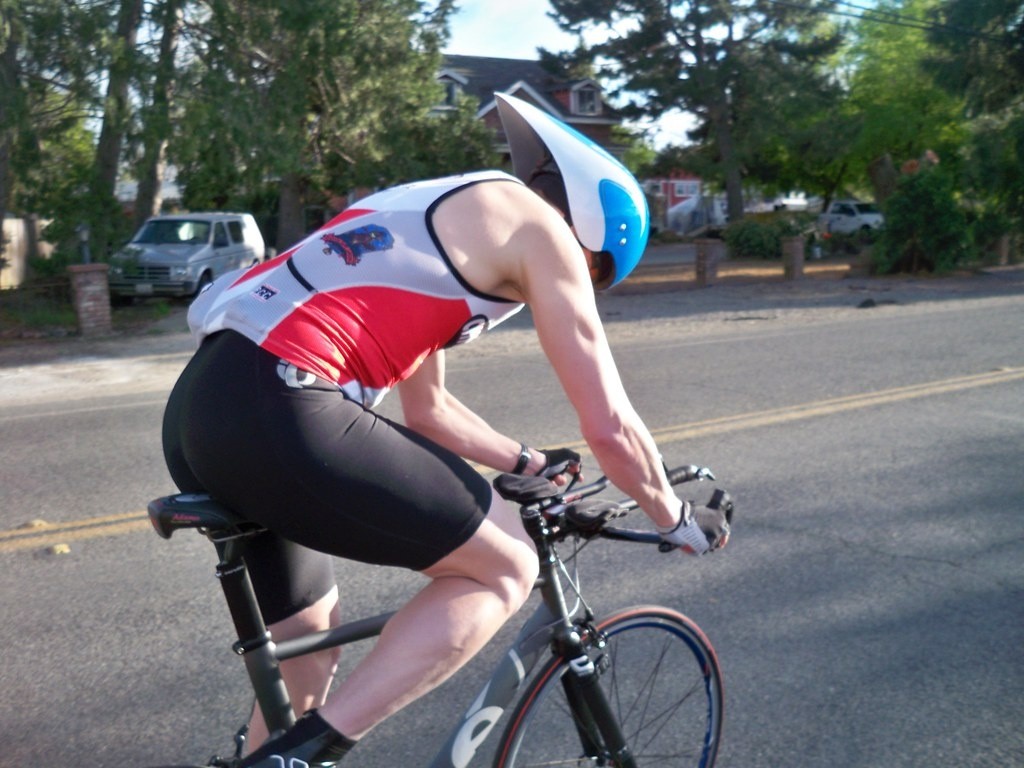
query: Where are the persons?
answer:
[161,92,732,768]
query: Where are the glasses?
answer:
[594,251,613,284]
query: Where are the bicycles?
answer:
[147,460,736,768]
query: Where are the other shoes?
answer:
[236,738,338,768]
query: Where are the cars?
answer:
[819,200,884,235]
[107,212,265,308]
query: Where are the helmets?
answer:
[494,91,650,291]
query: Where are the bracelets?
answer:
[511,443,531,475]
